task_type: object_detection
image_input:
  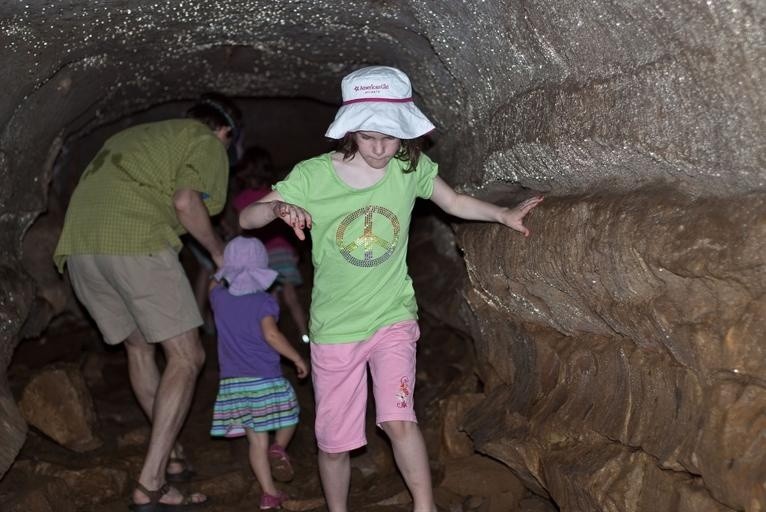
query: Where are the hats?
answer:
[322,66,438,141]
[209,234,279,296]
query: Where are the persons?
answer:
[239,60,546,511]
[205,232,311,510]
[50,87,252,512]
[179,149,310,353]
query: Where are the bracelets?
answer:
[293,357,303,363]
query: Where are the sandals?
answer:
[128,480,211,511]
[268,443,295,483]
[258,488,291,511]
[161,457,193,485]
